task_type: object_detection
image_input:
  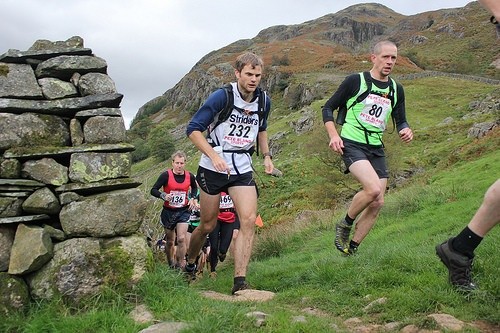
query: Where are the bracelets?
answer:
[160,192,168,200]
[262,152,272,159]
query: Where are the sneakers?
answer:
[436,236,476,294]
[334,218,353,254]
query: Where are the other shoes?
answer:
[219,254,226,262]
[231,282,248,294]
[184,261,197,279]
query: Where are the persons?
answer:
[434,0,500,303]
[322,40,414,259]
[182,52,275,297]
[155,173,241,284]
[150,151,198,274]
